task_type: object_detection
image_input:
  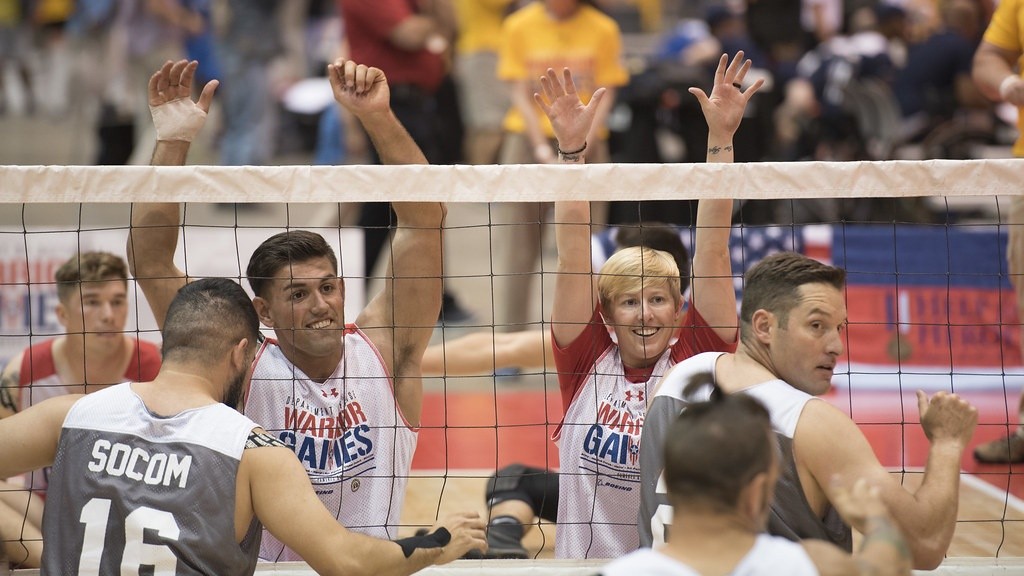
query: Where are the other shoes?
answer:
[418,523,529,559]
[975,433,1024,462]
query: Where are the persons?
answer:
[1,250,162,570]
[595,372,912,576]
[0,2,1024,470]
[413,463,558,560]
[532,48,764,562]
[125,56,449,568]
[638,251,980,571]
[1,277,488,576]
